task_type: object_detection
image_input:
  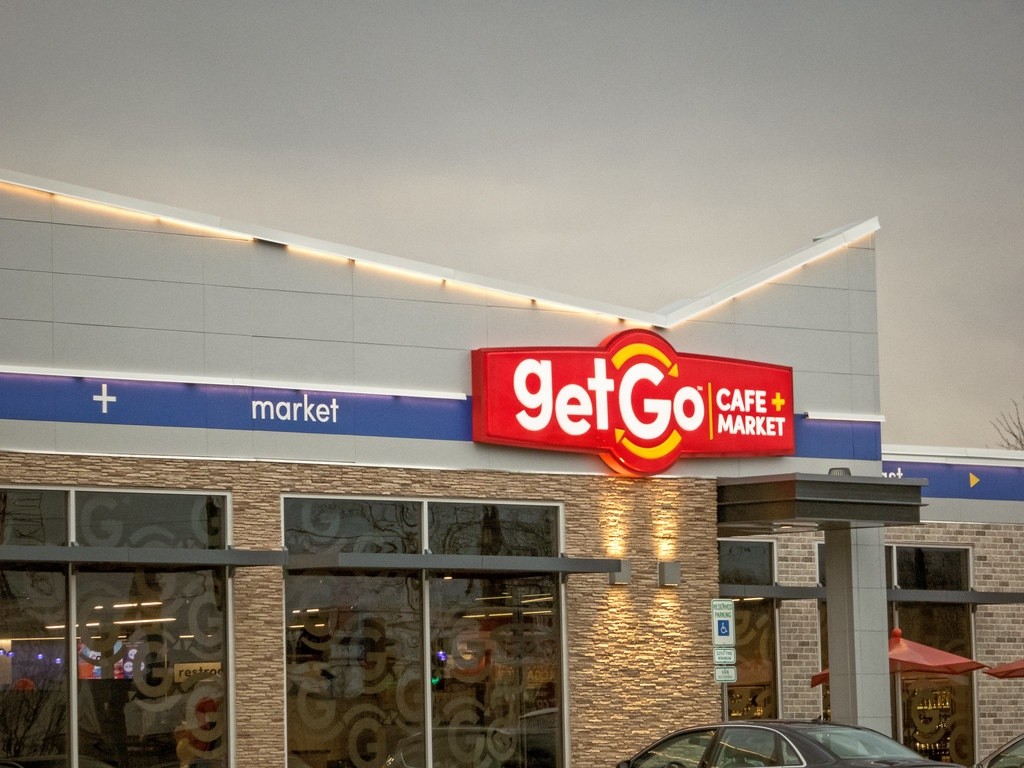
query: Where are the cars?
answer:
[442,606,561,707]
[976,733,1024,768]
[616,719,966,768]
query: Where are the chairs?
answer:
[723,739,755,768]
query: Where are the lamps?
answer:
[659,562,681,587]
[609,559,631,586]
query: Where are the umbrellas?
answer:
[982,658,1024,679]
[810,628,992,744]
